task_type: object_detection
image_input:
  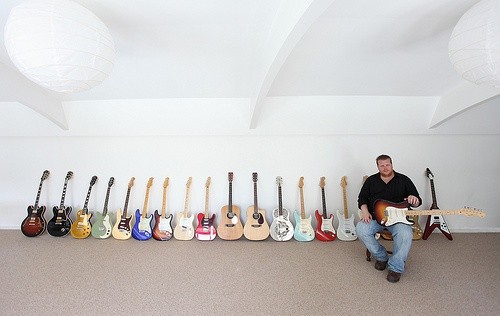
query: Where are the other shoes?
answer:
[375,260,386,270]
[387,270,400,282]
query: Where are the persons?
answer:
[355,155,422,283]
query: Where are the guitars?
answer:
[91,177,115,239]
[111,176,136,240]
[423,167,453,240]
[336,176,358,241]
[269,176,294,241]
[71,176,97,239]
[315,176,336,241]
[217,172,244,240]
[132,177,154,240]
[195,177,217,241]
[373,199,486,227]
[21,170,50,237]
[174,176,195,241]
[243,172,270,241]
[355,175,422,241]
[152,176,174,241]
[46,171,74,237]
[293,177,315,242]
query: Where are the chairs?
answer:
[365,229,393,262]
